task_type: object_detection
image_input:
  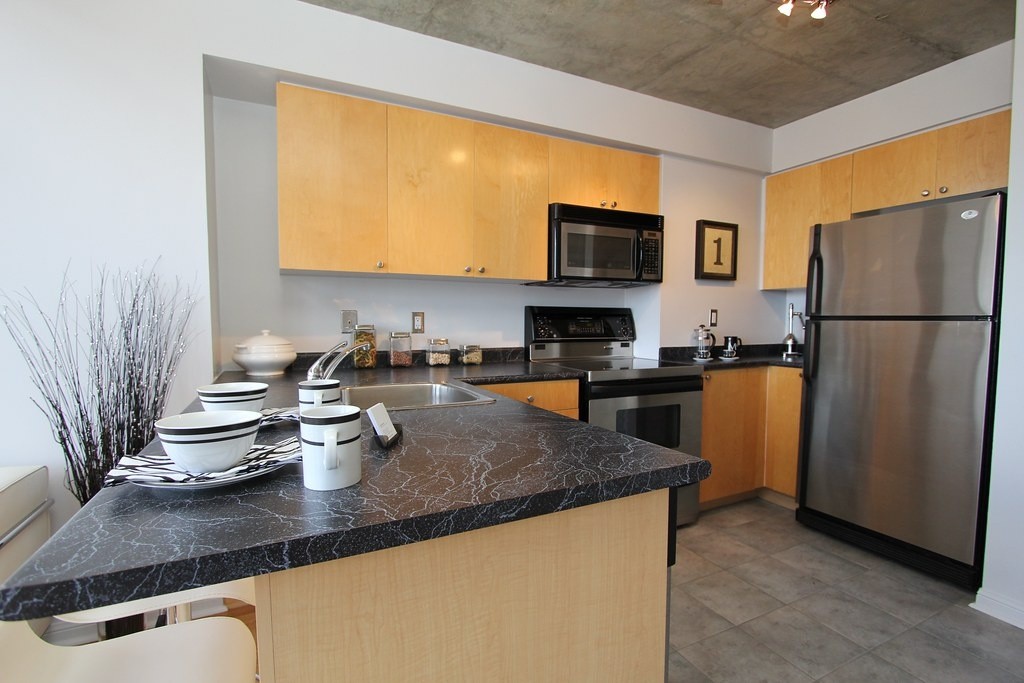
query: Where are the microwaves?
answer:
[520,202,664,290]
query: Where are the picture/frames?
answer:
[695,219,739,280]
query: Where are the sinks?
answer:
[340,382,496,414]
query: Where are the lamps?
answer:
[777,0,827,19]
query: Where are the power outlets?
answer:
[412,312,424,333]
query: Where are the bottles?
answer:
[463,344,483,365]
[353,324,376,369]
[693,324,717,359]
[390,332,413,367]
[428,337,451,367]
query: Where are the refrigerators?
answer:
[794,191,1007,591]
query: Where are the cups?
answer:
[297,379,341,414]
[723,335,742,358]
[722,349,736,358]
[299,404,363,491]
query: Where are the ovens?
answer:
[579,374,704,527]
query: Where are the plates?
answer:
[128,444,286,490]
[719,356,739,362]
[693,357,713,363]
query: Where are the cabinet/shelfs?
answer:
[764,108,1012,290]
[701,365,802,505]
[275,81,659,280]
[474,379,580,421]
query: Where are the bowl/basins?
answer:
[154,410,263,473]
[232,329,298,376]
[195,381,269,411]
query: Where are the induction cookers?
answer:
[524,305,705,383]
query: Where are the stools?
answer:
[1,577,256,683]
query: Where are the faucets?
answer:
[321,343,371,380]
[306,340,348,380]
